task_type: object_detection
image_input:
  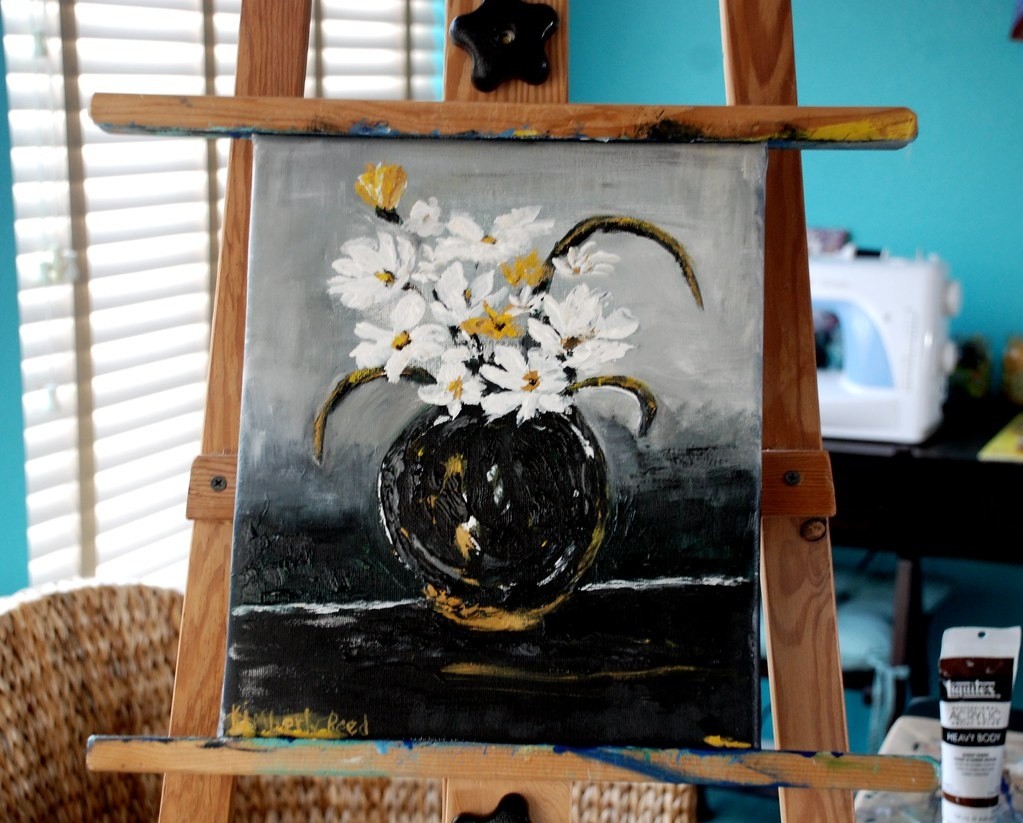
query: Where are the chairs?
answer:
[0,574,694,823]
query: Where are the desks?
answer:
[822,369,1023,729]
